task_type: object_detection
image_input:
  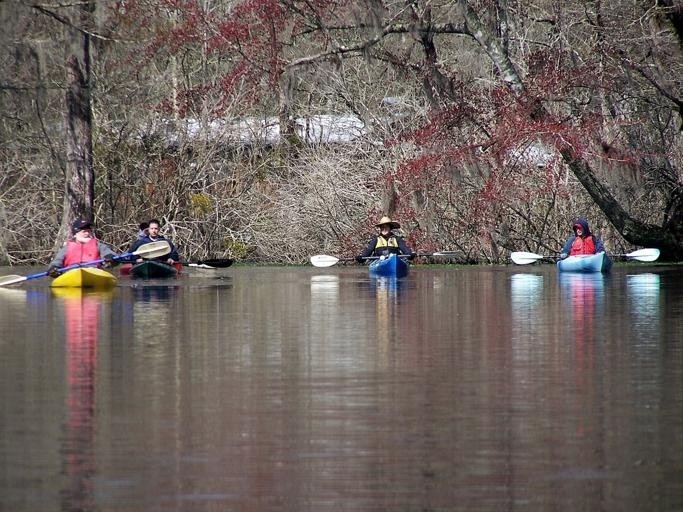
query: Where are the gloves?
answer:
[355,255,367,263]
[104,253,115,265]
[407,253,416,260]
[600,251,605,254]
[49,266,62,276]
[560,253,567,259]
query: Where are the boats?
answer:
[556,251,614,273]
[128,259,179,276]
[368,253,410,277]
[49,266,118,288]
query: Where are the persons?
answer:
[49,219,121,278]
[560,220,606,259]
[129,219,179,265]
[356,216,415,264]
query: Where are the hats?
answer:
[72,219,93,235]
[574,224,582,229]
[375,217,400,229]
[140,223,149,231]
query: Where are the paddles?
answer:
[311,250,466,270]
[511,248,660,266]
[0,240,172,290]
[116,259,234,268]
